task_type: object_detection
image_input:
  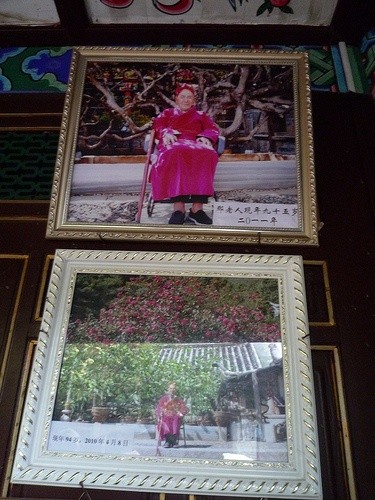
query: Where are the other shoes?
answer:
[164,441,172,447]
[167,438,178,444]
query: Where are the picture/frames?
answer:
[9,249,323,500]
[46,46,320,246]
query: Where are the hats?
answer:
[176,83,194,98]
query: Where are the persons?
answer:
[148,83,220,225]
[155,383,187,448]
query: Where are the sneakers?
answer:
[188,206,213,226]
[169,209,187,225]
